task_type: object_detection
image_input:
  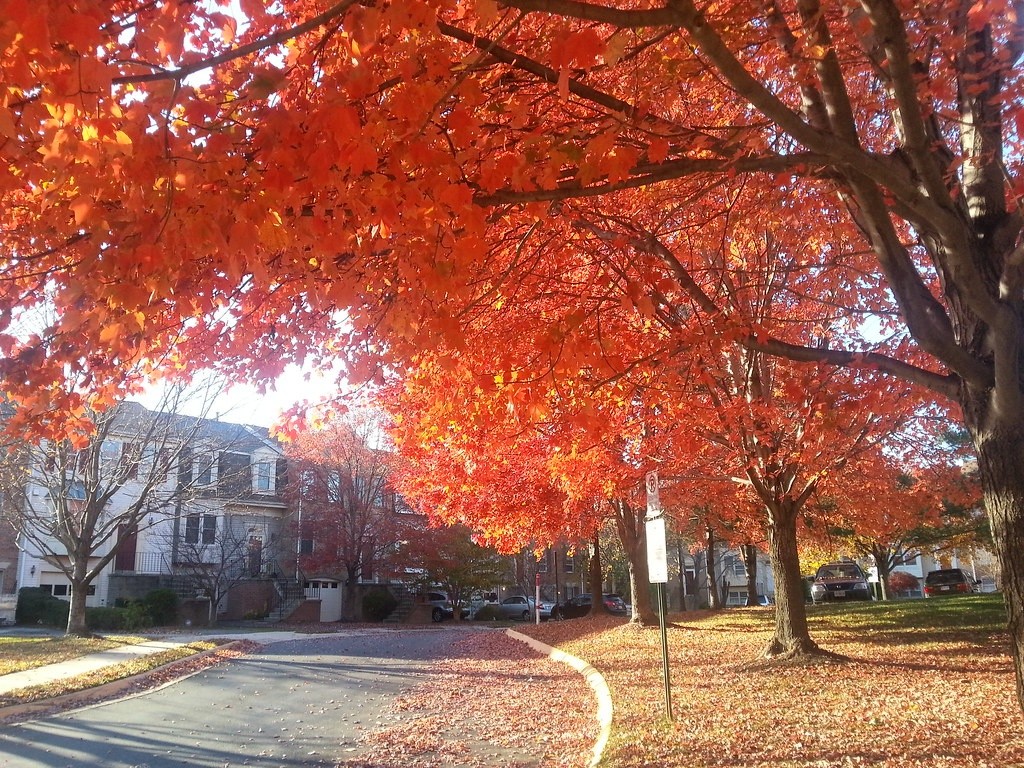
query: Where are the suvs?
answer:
[415,591,471,623]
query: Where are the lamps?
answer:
[30,564,36,578]
[148,515,154,530]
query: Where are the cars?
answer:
[807,560,873,605]
[922,568,982,598]
[550,593,628,621]
[497,593,554,623]
